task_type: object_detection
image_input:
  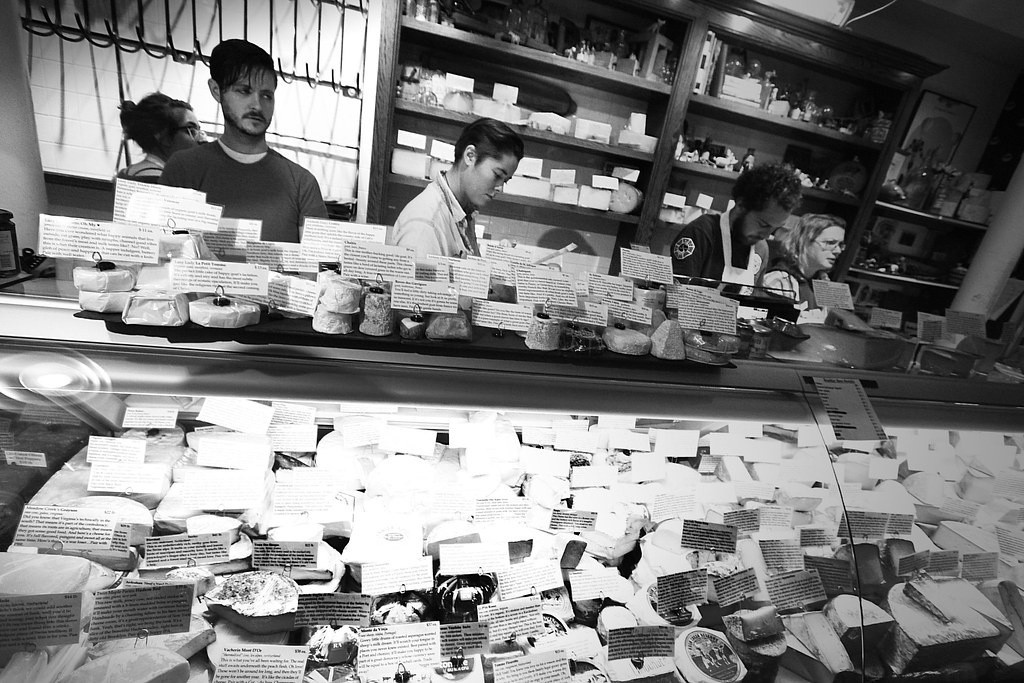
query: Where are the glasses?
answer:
[811,240,847,251]
[166,125,207,142]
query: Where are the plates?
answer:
[682,340,738,367]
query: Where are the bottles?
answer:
[931,184,948,215]
[738,147,756,173]
[0,208,21,278]
[852,229,872,269]
[404,0,439,24]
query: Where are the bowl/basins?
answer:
[759,320,810,350]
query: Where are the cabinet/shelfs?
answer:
[357,0,990,321]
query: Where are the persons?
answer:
[761,214,846,323]
[391,118,525,261]
[670,160,803,296]
[157,38,329,244]
[113,92,208,185]
